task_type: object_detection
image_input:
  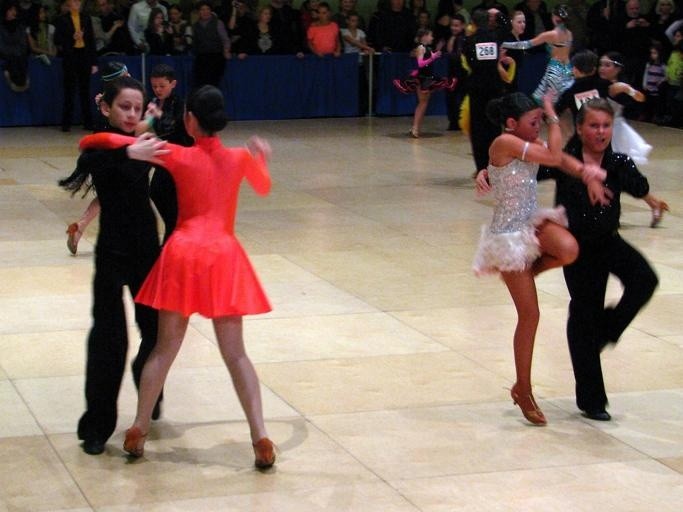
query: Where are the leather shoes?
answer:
[584,409,610,421]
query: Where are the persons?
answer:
[59,64,275,469]
[1,0,682,133]
[472,49,670,427]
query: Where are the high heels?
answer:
[251,438,275,468]
[123,428,147,457]
[510,384,546,426]
[66,223,81,254]
[650,201,668,226]
